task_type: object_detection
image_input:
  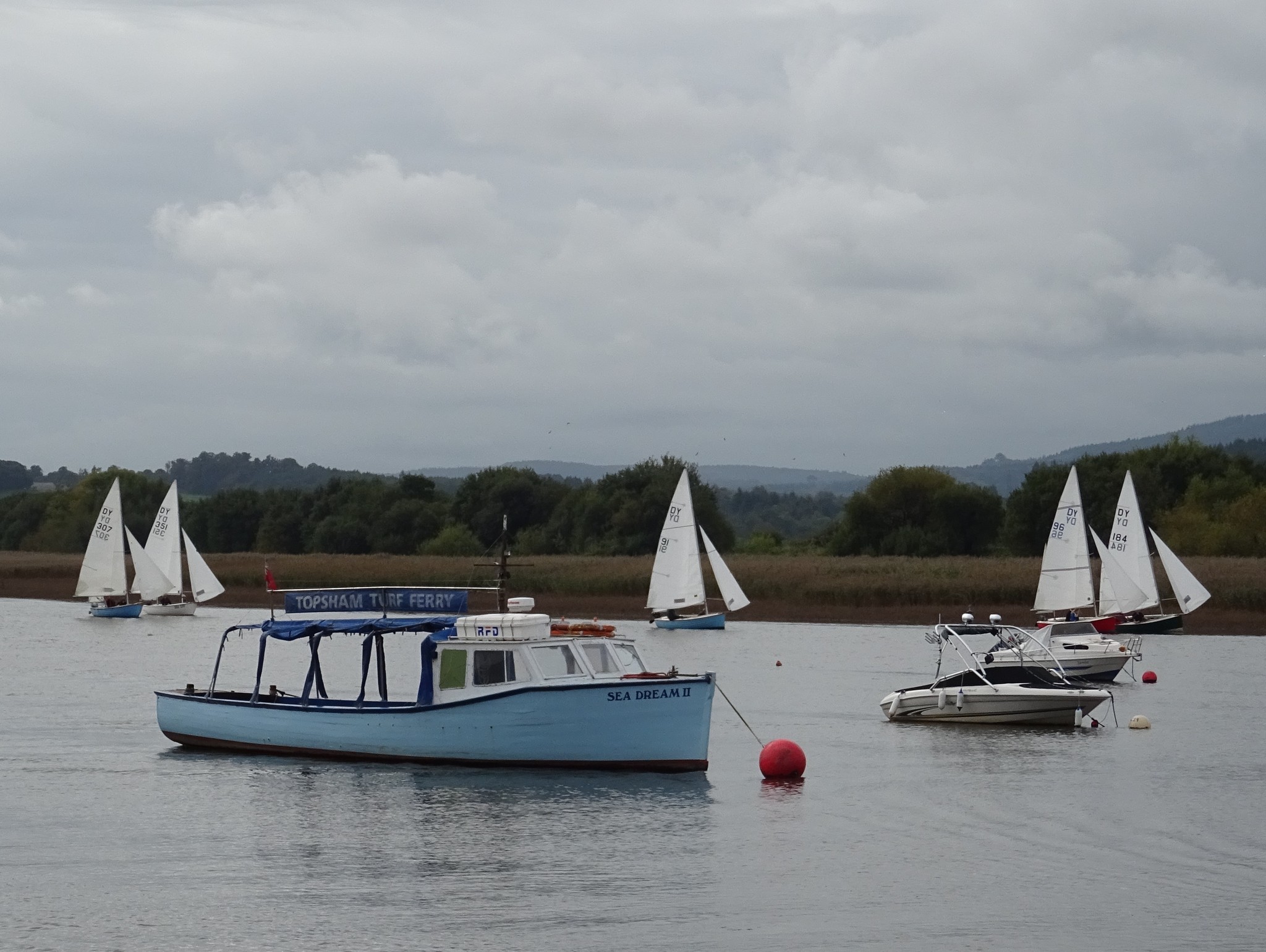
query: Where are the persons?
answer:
[160,595,171,605]
[697,609,705,616]
[667,609,687,621]
[1070,608,1080,622]
[117,598,127,606]
[1132,610,1150,623]
[105,596,115,607]
[1065,608,1076,622]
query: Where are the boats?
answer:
[976,619,1143,683]
[877,611,1114,727]
[154,519,717,775]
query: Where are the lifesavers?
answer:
[550,624,616,632]
[551,630,615,637]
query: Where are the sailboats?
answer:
[1096,469,1212,635]
[642,468,751,630]
[1030,465,1148,633]
[129,477,226,617]
[71,476,178,618]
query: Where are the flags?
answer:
[264,566,277,590]
